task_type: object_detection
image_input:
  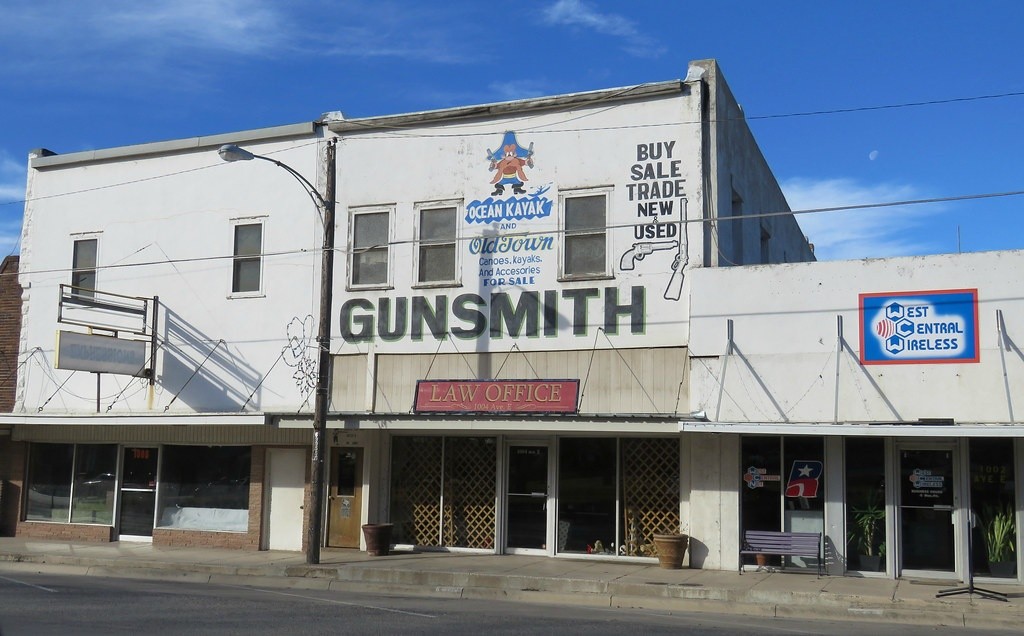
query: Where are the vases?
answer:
[362,522,394,556]
[754,554,772,566]
[653,534,689,568]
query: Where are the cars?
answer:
[80,473,149,498]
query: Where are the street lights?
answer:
[218,144,335,564]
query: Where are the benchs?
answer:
[739,529,822,579]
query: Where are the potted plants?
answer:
[847,504,886,570]
[973,507,1015,578]
